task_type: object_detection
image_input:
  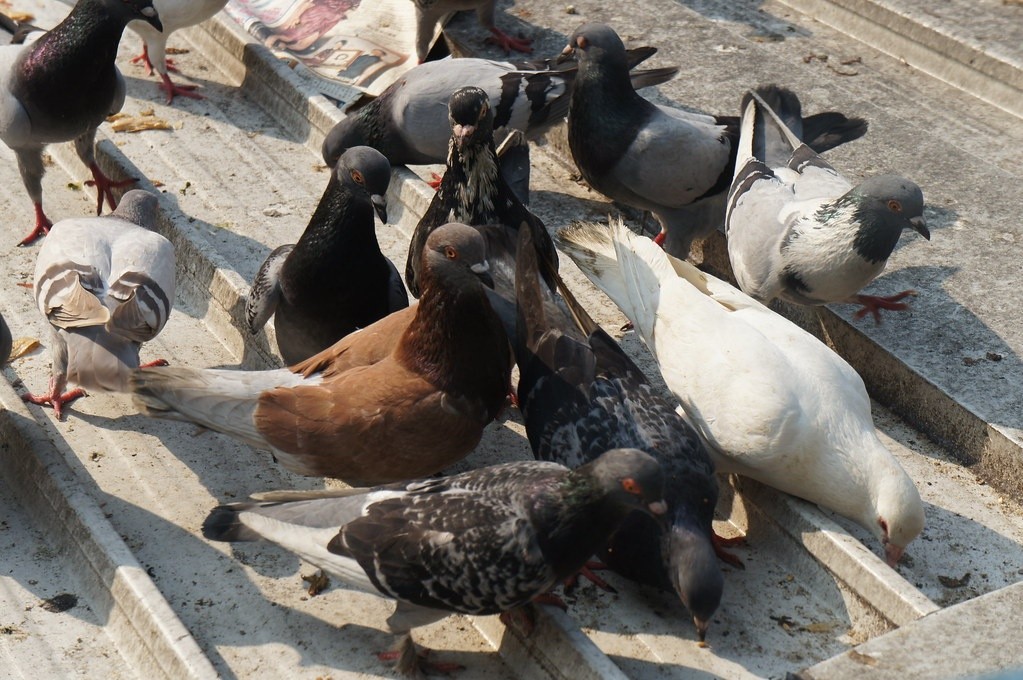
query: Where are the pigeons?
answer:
[0,0,229,420]
[140,0,923,675]
